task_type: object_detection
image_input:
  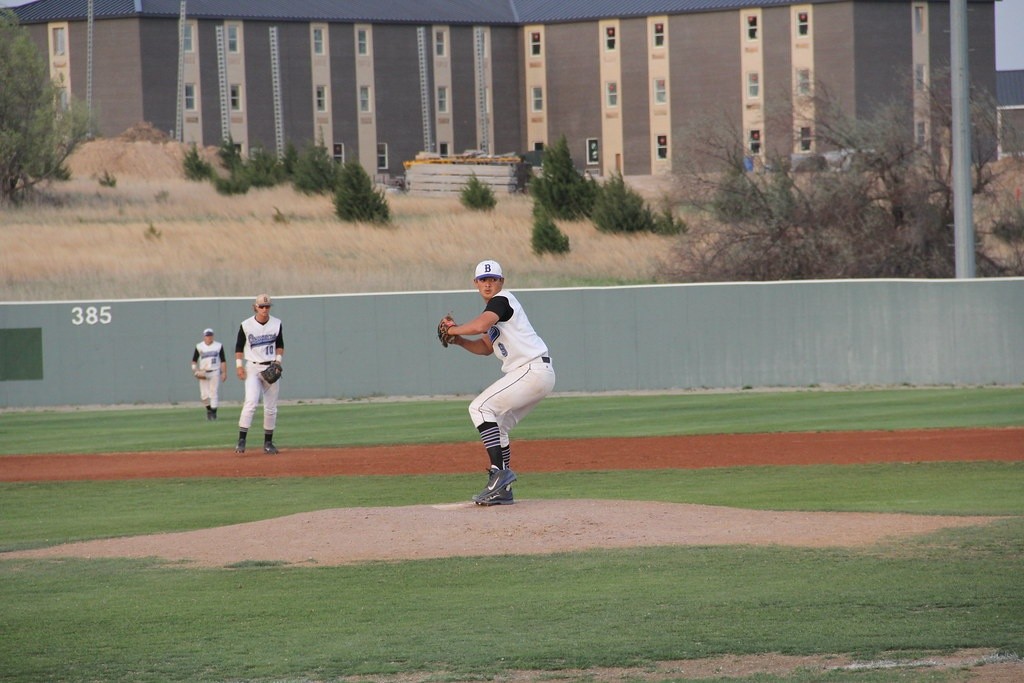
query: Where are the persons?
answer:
[234,293,285,456]
[191,328,227,421]
[436,260,557,507]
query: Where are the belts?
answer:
[206,370,212,372]
[253,362,270,365]
[542,357,550,363]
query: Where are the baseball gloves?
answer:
[194,369,207,379]
[258,362,283,385]
[437,316,457,348]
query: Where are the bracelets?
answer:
[192,365,196,370]
[275,354,283,362]
[236,359,243,368]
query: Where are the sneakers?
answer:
[471,487,514,504]
[477,467,516,499]
[235,438,246,453]
[263,441,278,454]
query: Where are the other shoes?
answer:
[212,412,216,418]
[208,410,212,419]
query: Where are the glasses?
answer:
[258,305,270,309]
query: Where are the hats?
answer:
[256,294,270,306]
[203,329,214,336]
[475,260,502,279]
